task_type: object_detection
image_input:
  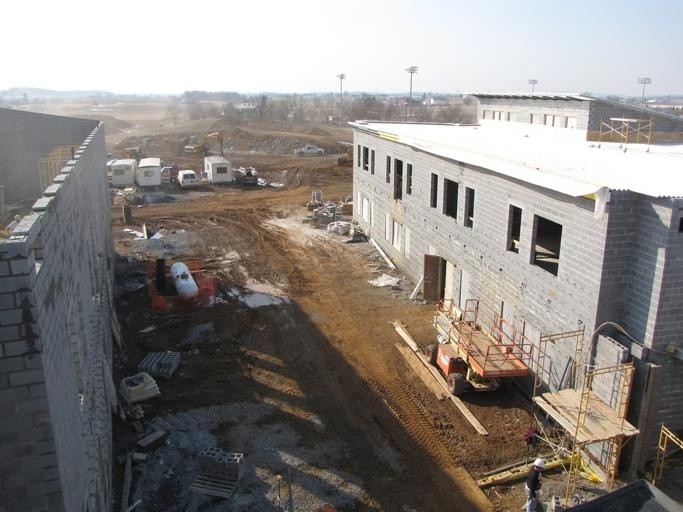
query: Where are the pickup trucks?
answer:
[295,146,325,156]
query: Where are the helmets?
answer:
[14,215,20,220]
[535,458,545,469]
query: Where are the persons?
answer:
[5,214,22,239]
[521,457,545,512]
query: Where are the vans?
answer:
[177,170,199,188]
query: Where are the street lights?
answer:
[528,79,538,91]
[637,76,652,108]
[337,73,344,128]
[405,66,417,121]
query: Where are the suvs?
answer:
[235,166,258,185]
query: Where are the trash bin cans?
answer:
[122,205,132,224]
